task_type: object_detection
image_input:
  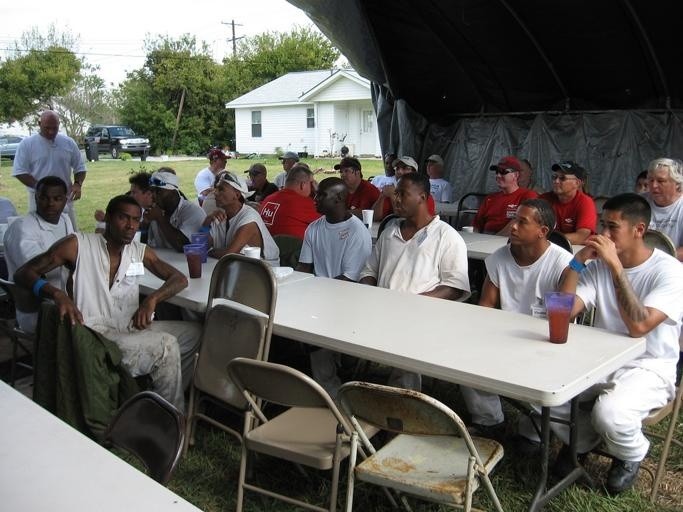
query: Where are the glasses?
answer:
[495,168,514,175]
[149,177,178,187]
[249,172,263,176]
[551,174,575,181]
[392,166,413,171]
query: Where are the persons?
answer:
[424,155,452,204]
[273,152,300,188]
[201,171,231,215]
[371,153,398,191]
[181,167,280,321]
[459,196,574,447]
[517,160,544,193]
[12,194,204,428]
[371,156,435,222]
[467,155,537,283]
[577,167,593,198]
[556,194,683,496]
[334,157,380,218]
[195,150,231,207]
[9,110,86,230]
[301,173,470,418]
[539,161,597,246]
[642,158,682,259]
[3,177,77,334]
[634,170,650,194]
[292,162,309,169]
[97,168,208,252]
[243,164,279,208]
[259,166,323,240]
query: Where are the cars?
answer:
[0,133,27,161]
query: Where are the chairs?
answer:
[0,174,683,511]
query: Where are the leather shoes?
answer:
[468,421,587,478]
[607,459,639,492]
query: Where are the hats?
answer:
[489,157,520,170]
[278,151,300,162]
[392,156,418,172]
[216,170,256,199]
[427,154,444,165]
[334,158,361,170]
[244,163,267,174]
[149,172,179,191]
[551,160,582,175]
[208,149,231,159]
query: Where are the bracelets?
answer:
[141,221,151,231]
[207,247,213,253]
[569,259,586,274]
[75,181,82,188]
[31,278,48,295]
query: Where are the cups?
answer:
[242,246,262,260]
[0,215,20,247]
[134,231,141,242]
[183,232,208,278]
[544,291,574,343]
[362,210,374,229]
[462,226,474,235]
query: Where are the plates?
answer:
[271,267,293,279]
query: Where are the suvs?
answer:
[83,123,151,160]
